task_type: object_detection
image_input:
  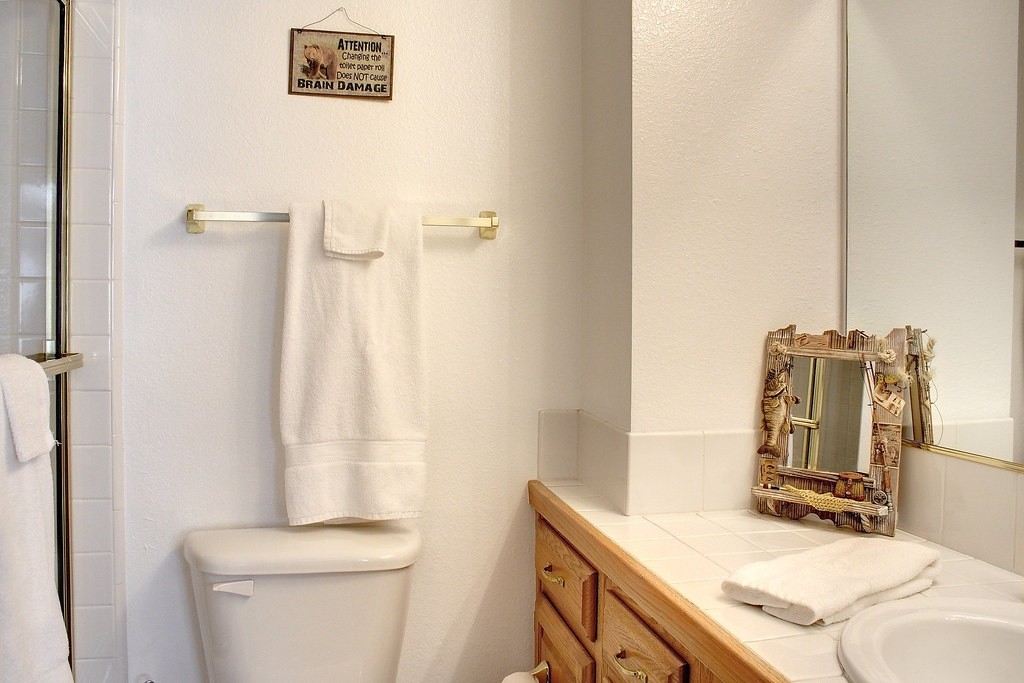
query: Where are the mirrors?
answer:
[845,0,1024,472]
[750,324,906,536]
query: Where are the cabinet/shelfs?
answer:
[500,481,790,683]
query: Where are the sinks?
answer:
[837,591,1022,683]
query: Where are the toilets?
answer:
[180,528,423,683]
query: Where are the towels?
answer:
[723,535,943,625]
[278,193,429,526]
[0,353,73,682]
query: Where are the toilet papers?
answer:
[499,670,541,683]
